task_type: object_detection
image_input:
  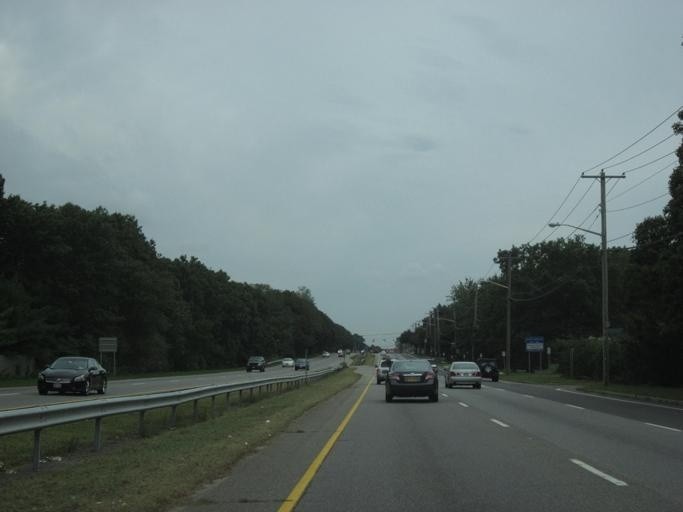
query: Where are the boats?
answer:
[321,347,399,363]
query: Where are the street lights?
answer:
[548,222,611,390]
[411,316,459,359]
[479,279,512,375]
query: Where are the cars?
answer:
[282,356,294,368]
[34,354,109,399]
[374,360,440,402]
[443,360,483,390]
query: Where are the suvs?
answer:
[295,358,310,371]
[246,355,266,372]
[474,357,501,382]
